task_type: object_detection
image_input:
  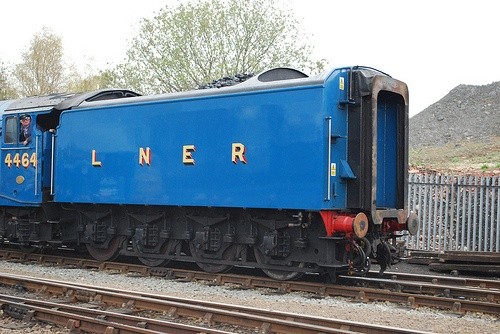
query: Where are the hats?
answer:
[18,114,27,119]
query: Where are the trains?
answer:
[0,64,420,285]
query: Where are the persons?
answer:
[19,114,32,146]
